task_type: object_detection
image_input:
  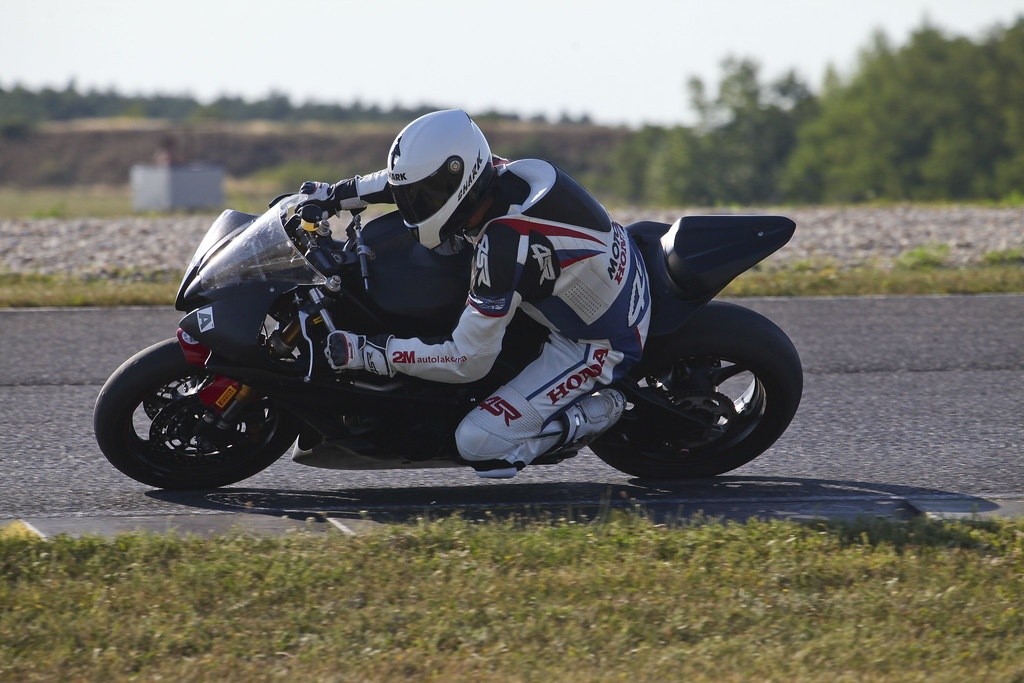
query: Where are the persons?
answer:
[298,109,655,478]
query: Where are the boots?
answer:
[543,387,626,461]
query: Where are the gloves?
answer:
[322,329,396,378]
[299,175,367,228]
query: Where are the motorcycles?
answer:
[93,188,804,492]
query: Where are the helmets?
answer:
[387,109,493,250]
